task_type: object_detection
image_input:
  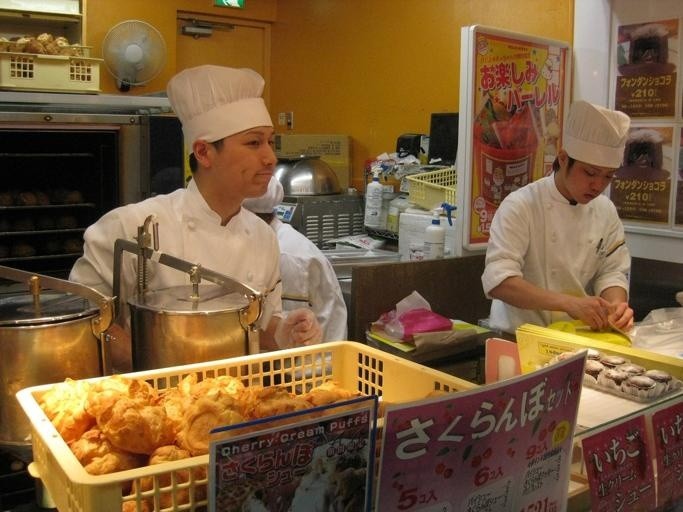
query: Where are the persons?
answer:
[240,175,347,395]
[68,65,323,374]
[479,99,637,334]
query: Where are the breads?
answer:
[0,33,82,78]
[41,371,449,512]
[559,347,672,399]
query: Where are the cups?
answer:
[625,139,664,169]
[629,36,670,64]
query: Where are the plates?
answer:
[617,63,676,75]
[614,166,671,180]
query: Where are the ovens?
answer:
[0,110,186,296]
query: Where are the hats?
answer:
[166,65,273,156]
[562,100,631,169]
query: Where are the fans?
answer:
[100,18,170,89]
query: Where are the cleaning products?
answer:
[364,165,388,226]
[423,198,458,258]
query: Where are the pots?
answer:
[1,286,110,453]
[125,278,269,393]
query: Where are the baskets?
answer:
[0,41,105,94]
[405,167,456,219]
[15,340,482,512]
